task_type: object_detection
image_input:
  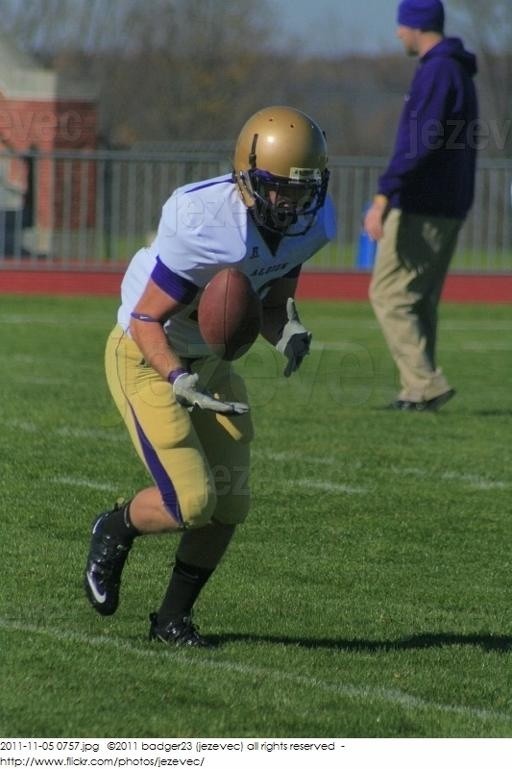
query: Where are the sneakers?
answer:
[149,612,211,647]
[83,502,132,615]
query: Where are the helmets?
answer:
[232,106,330,236]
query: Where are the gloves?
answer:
[275,298,311,376]
[173,373,250,415]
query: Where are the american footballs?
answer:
[199,268,261,362]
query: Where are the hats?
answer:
[398,0,444,31]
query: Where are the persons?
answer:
[82,103,338,649]
[362,0,480,411]
